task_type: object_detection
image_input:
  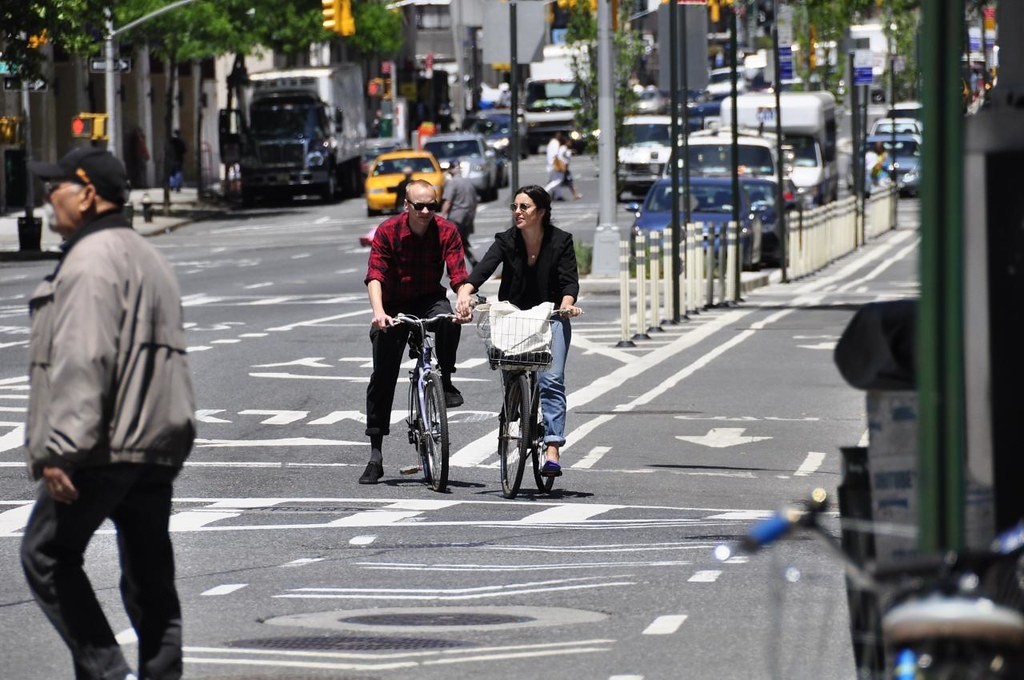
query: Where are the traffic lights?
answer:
[72,112,95,141]
[368,78,386,97]
[322,0,356,37]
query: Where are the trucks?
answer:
[219,60,368,207]
[518,41,596,154]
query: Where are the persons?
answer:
[359,179,469,483]
[125,128,148,189]
[865,142,886,194]
[20,143,199,680]
[394,167,412,214]
[163,129,186,191]
[372,109,381,138]
[442,160,478,268]
[456,186,580,476]
[543,133,583,202]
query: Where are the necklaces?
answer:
[532,255,535,258]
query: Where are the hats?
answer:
[31,146,131,206]
[449,160,460,169]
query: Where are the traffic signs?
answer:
[89,57,131,74]
[4,75,49,93]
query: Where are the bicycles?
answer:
[731,484,1024,680]
[383,312,456,493]
[467,293,585,501]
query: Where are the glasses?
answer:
[405,198,438,210]
[42,182,83,196]
[510,203,537,211]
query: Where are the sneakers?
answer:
[442,380,464,408]
[359,457,384,484]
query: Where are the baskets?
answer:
[772,510,918,679]
[480,315,556,372]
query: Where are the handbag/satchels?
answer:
[553,160,567,173]
[474,300,555,356]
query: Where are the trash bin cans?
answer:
[17,217,43,252]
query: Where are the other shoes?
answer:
[110,671,138,680]
[498,393,519,421]
[542,460,561,472]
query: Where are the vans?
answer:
[718,89,840,210]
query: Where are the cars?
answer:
[462,21,997,282]
[361,150,451,217]
[360,135,415,176]
[419,130,498,203]
